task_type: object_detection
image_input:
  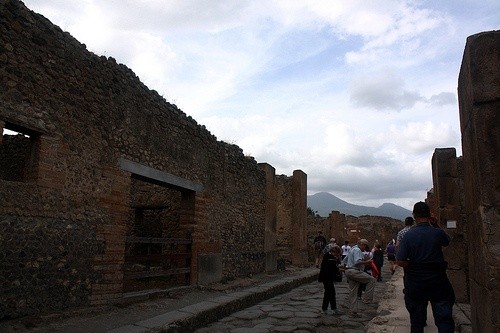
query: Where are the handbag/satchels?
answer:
[370,261,379,278]
[337,269,342,282]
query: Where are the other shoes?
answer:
[365,299,377,304]
[350,313,362,318]
[332,310,344,315]
[357,297,362,300]
[377,279,383,282]
[321,310,333,315]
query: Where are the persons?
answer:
[342,244,358,268]
[318,245,342,315]
[371,239,382,254]
[396,217,415,294]
[395,201,456,333]
[373,243,383,282]
[386,240,395,275]
[358,244,373,299]
[312,231,327,268]
[323,237,342,254]
[343,239,377,308]
[340,241,351,260]
[386,239,397,245]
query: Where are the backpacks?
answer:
[376,252,383,267]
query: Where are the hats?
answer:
[360,239,369,246]
[330,238,335,242]
[405,217,415,223]
[413,202,430,215]
